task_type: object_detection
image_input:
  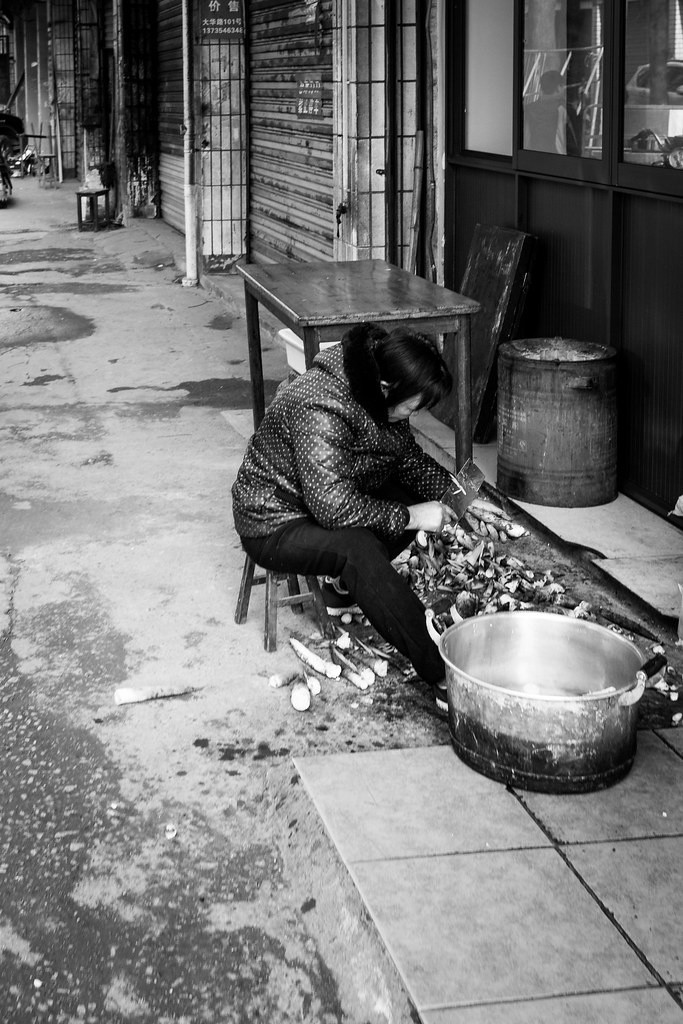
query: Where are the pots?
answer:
[422,597,667,796]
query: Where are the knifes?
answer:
[415,458,486,548]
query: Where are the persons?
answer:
[524,72,580,156]
[230,323,512,712]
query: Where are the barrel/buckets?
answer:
[493,335,619,506]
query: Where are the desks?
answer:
[233,257,480,484]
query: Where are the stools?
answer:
[234,553,338,653]
[39,153,58,189]
[75,186,110,230]
